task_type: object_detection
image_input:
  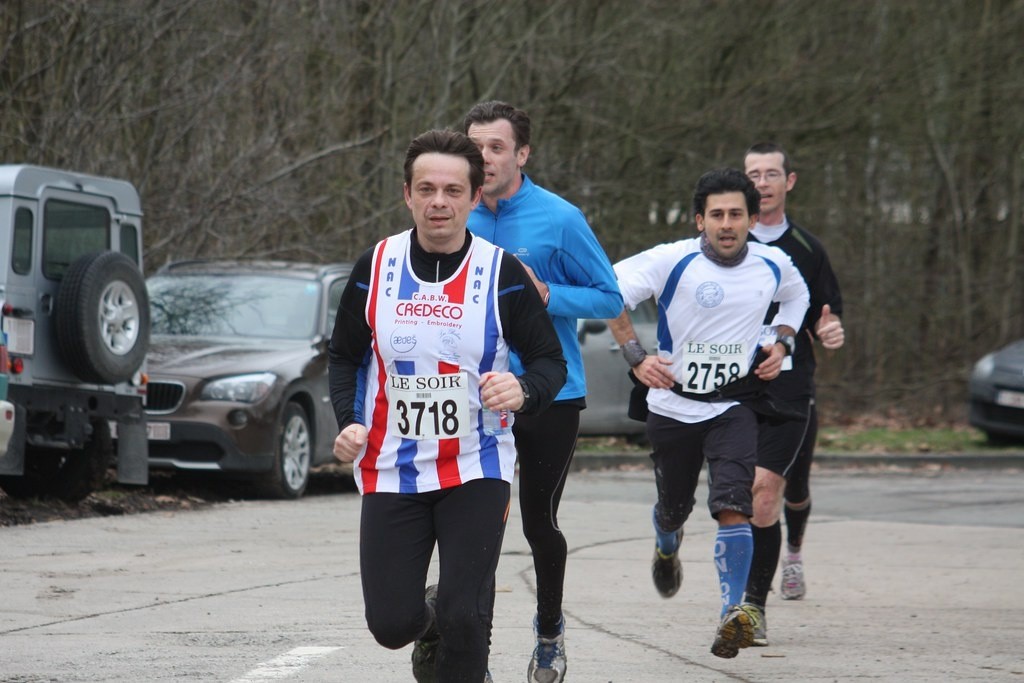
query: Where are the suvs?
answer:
[0,160,154,500]
[109,252,359,503]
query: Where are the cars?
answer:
[967,338,1024,448]
[574,297,659,450]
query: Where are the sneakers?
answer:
[711,603,760,658]
[752,607,769,646]
[780,558,806,600]
[411,584,439,683]
[527,612,567,683]
[484,669,494,683]
[652,525,685,598]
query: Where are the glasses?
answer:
[751,171,786,183]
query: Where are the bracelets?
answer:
[620,341,647,366]
[544,293,550,307]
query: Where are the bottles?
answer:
[480,374,512,437]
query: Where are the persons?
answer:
[606,168,810,658]
[326,131,568,683]
[464,101,624,683]
[731,142,844,646]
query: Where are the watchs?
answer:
[775,334,797,358]
